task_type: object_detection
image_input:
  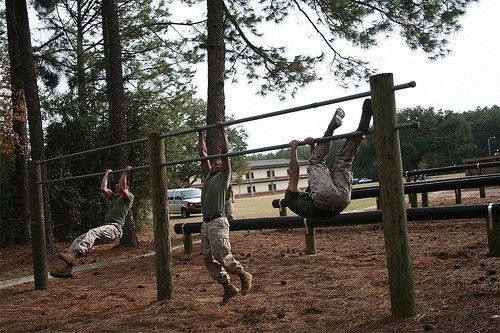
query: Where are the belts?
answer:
[203,213,226,223]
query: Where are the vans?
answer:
[167,188,202,218]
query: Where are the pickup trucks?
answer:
[358,178,373,184]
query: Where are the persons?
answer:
[194,119,253,307]
[283,98,372,222]
[49,165,134,277]
[225,184,234,221]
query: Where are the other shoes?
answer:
[58,250,81,268]
[359,99,372,131]
[220,280,239,306]
[328,107,345,131]
[50,264,73,278]
[237,269,252,296]
